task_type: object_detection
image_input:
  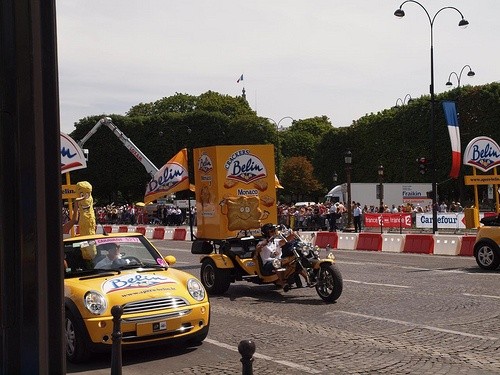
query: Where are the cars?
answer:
[65,232,212,364]
[472,213,500,272]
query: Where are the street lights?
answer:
[444,64,476,204]
[344,148,353,228]
[377,163,385,212]
[394,0,470,235]
[262,116,296,181]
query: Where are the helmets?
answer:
[261,223,277,239]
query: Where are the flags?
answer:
[442,102,461,179]
[237,74,243,83]
[144,151,190,205]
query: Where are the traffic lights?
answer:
[418,157,427,175]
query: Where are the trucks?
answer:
[324,183,433,213]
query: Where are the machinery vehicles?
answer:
[77,117,196,225]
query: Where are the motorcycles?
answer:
[192,223,344,303]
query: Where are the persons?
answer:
[255,223,317,292]
[62,202,78,234]
[277,201,362,233]
[424,201,464,212]
[363,202,423,229]
[94,243,130,269]
[63,204,197,225]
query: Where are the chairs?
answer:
[257,254,276,276]
[228,238,260,255]
[93,244,108,266]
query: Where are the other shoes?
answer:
[283,283,289,293]
[306,282,316,288]
